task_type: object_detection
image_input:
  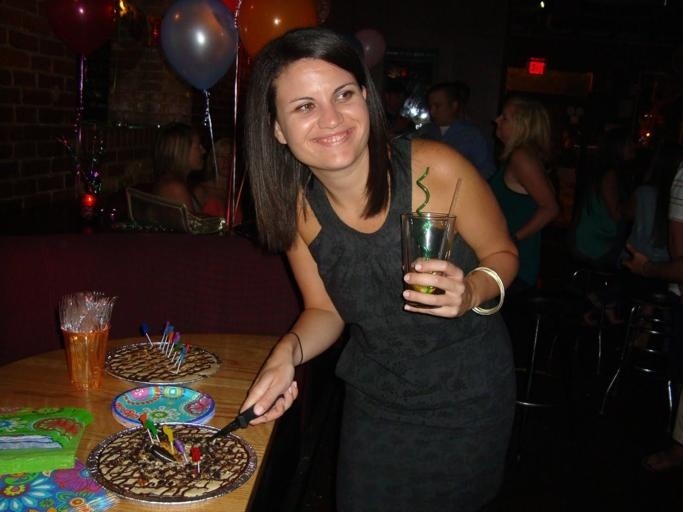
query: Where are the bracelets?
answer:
[463,265,505,317]
[286,332,302,365]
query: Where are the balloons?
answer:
[104,2,147,76]
[157,1,238,93]
[46,1,121,61]
[355,28,387,68]
[236,1,318,58]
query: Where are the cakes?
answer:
[99,427,249,497]
[109,345,221,383]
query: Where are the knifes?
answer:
[205,394,283,445]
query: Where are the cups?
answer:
[60,322,111,391]
[399,212,456,308]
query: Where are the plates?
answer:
[85,422,257,512]
[104,341,222,387]
[111,384,215,429]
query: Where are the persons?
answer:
[485,97,560,288]
[567,124,683,477]
[415,82,499,184]
[146,122,205,216]
[194,137,245,226]
[235,25,522,509]
